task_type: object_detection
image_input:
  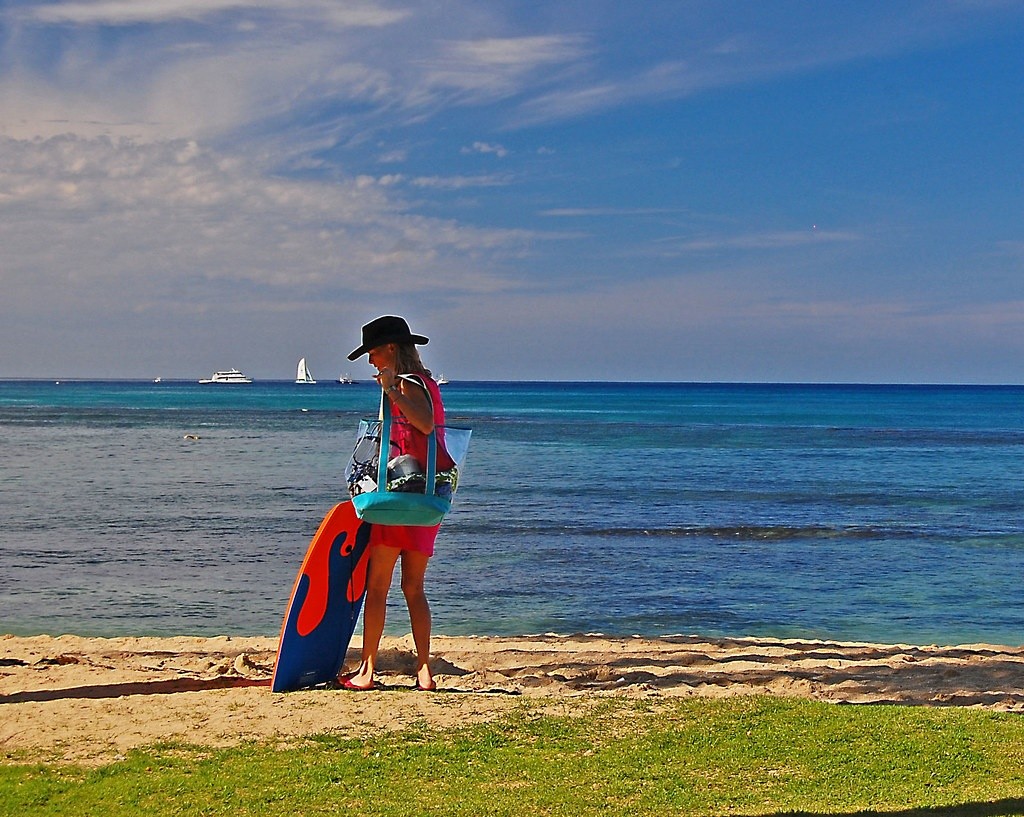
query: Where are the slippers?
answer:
[326,676,374,690]
[416,680,436,691]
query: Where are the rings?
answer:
[380,372,383,375]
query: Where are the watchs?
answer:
[385,385,397,395]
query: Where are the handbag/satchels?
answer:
[345,374,472,527]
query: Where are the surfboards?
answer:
[271,498,372,692]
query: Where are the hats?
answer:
[347,316,429,361]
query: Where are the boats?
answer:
[198,368,253,385]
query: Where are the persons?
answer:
[337,315,446,691]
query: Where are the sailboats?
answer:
[294,357,317,384]
[336,372,353,385]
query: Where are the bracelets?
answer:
[393,395,404,404]
